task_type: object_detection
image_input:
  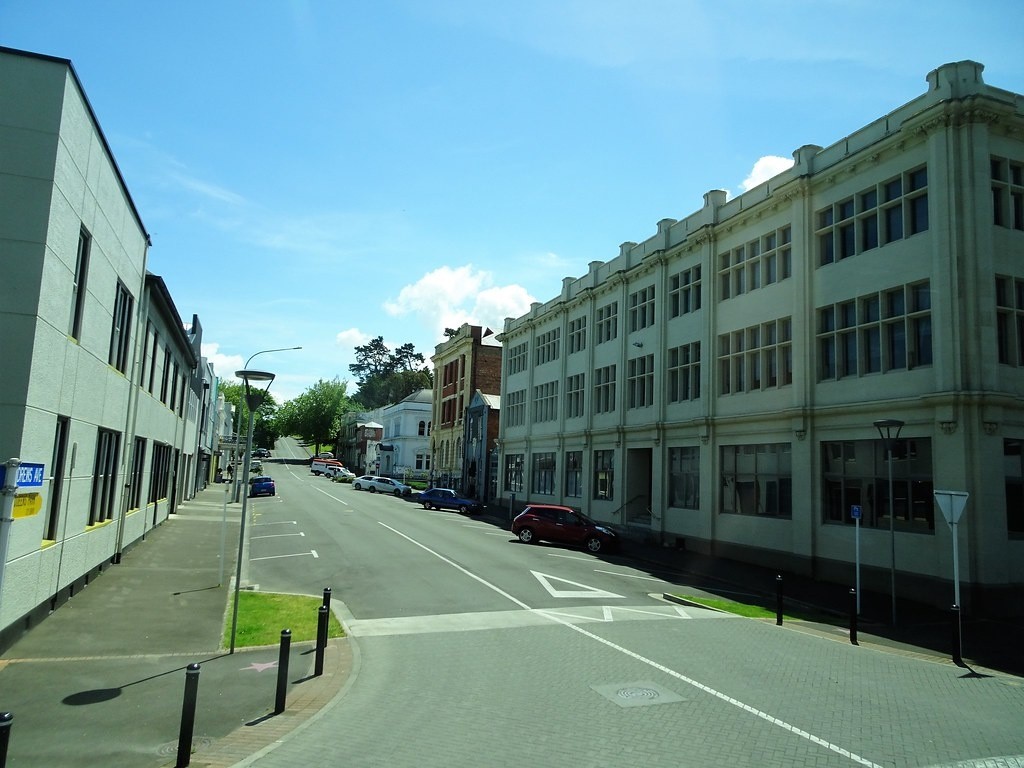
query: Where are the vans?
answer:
[310,459,343,476]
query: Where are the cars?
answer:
[510,505,619,554]
[249,475,276,498]
[417,488,482,515]
[249,460,264,473]
[352,475,377,491]
[314,452,335,459]
[368,477,411,497]
[324,465,356,478]
[251,448,272,458]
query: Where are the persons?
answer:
[227,464,233,479]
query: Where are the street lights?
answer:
[232,347,303,502]
[873,418,904,637]
[230,370,275,655]
